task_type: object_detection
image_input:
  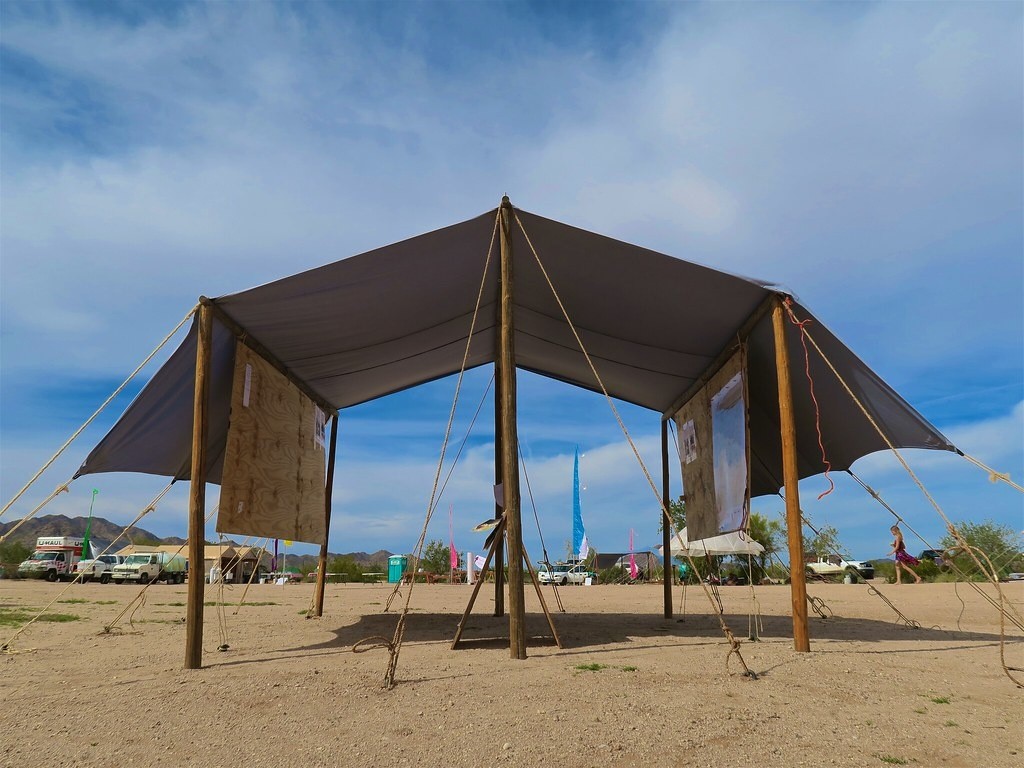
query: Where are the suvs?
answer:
[918,549,952,574]
[537,564,589,586]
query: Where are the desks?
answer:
[260,573,304,585]
[326,573,348,587]
[402,572,435,586]
[454,571,481,583]
[308,573,330,582]
[362,572,385,586]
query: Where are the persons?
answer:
[887,518,921,584]
[727,573,739,584]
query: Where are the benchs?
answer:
[433,576,461,585]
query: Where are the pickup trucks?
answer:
[805,554,874,583]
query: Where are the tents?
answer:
[73,197,963,670]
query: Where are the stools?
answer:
[455,577,462,585]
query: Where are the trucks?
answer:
[112,552,187,585]
[17,537,91,582]
[68,554,128,584]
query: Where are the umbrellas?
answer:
[660,522,766,586]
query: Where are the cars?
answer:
[619,562,642,578]
